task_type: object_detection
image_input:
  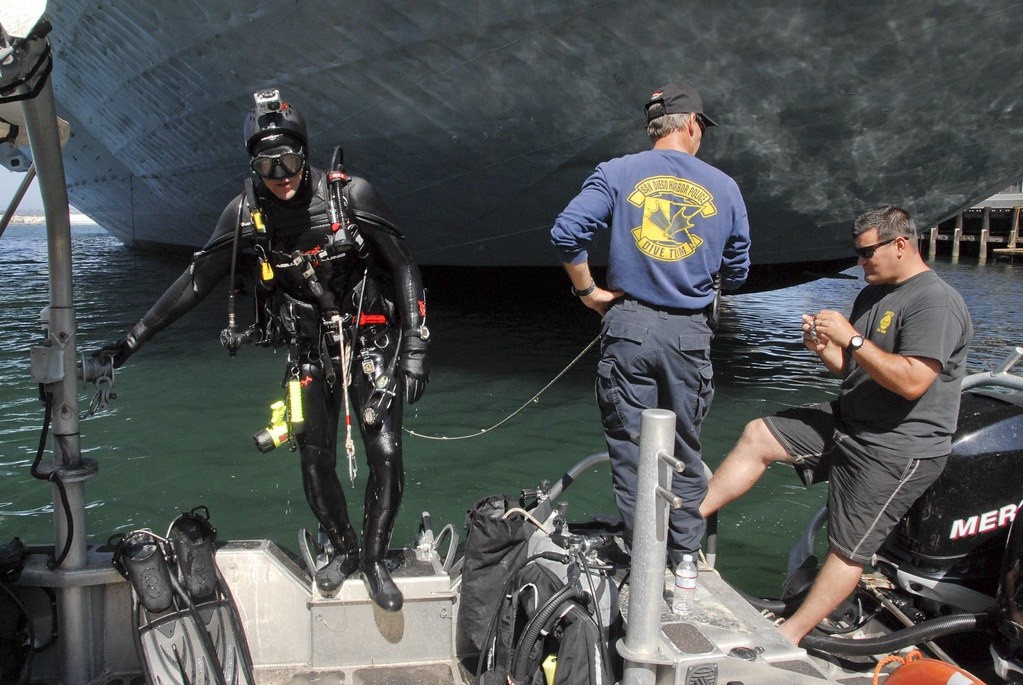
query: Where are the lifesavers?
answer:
[871,649,988,685]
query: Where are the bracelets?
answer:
[571,279,596,296]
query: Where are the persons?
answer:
[550,85,750,577]
[90,87,432,612]
[696,204,972,652]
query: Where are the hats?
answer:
[644,83,719,128]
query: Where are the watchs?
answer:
[403,325,431,341]
[846,335,865,353]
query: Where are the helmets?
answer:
[251,151,305,177]
[243,87,309,158]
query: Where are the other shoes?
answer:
[360,559,403,611]
[315,552,360,591]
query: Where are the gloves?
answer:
[398,337,431,404]
[92,333,139,368]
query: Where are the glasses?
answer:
[854,236,909,258]
